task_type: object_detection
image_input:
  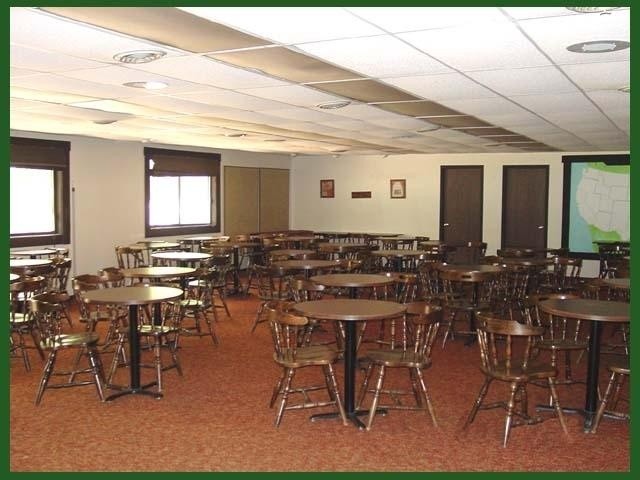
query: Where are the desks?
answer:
[537,298,630,432]
[80,287,184,402]
[295,299,408,432]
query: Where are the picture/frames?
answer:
[390,178,406,199]
[319,177,336,199]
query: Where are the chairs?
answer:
[463,310,568,450]
[522,292,603,406]
[9,275,46,372]
[591,357,630,434]
[107,282,183,391]
[262,299,349,428]
[28,289,107,407]
[9,228,629,371]
[355,301,443,431]
